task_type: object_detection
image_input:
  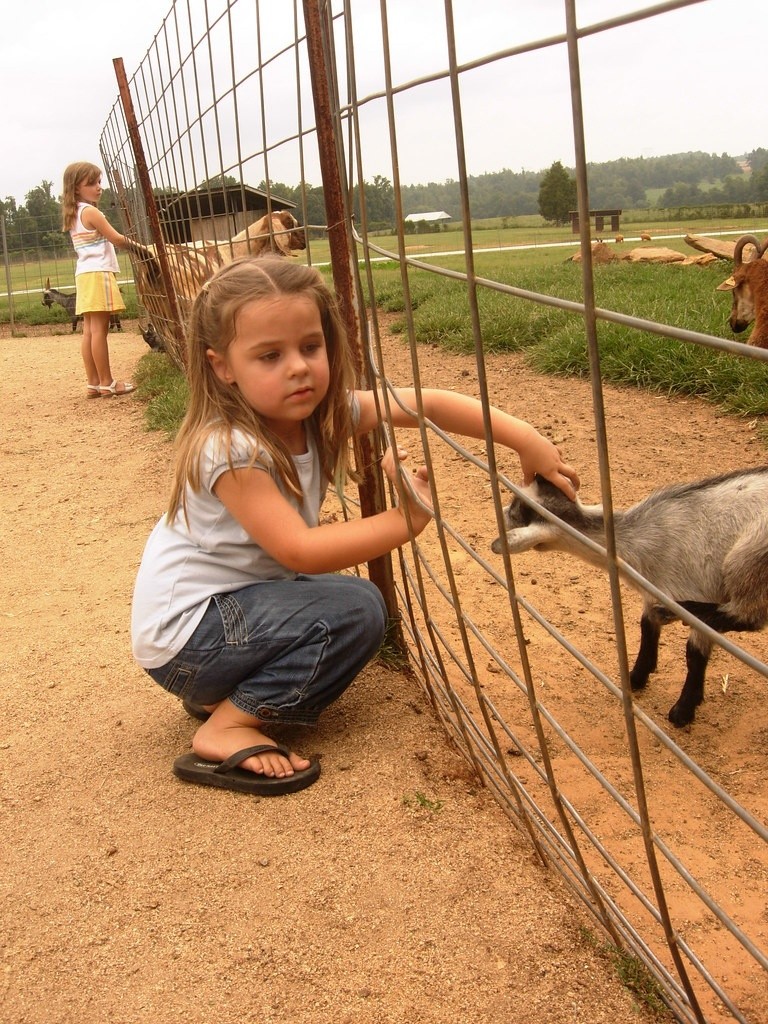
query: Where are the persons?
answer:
[128,256,581,799]
[62,161,153,401]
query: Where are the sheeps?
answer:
[42,278,125,333]
[716,234,768,350]
[139,211,307,353]
[595,234,652,243]
[491,463,768,727]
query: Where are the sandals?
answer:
[99,379,138,398]
[87,382,100,399]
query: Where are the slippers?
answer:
[180,700,214,722]
[173,739,322,796]
